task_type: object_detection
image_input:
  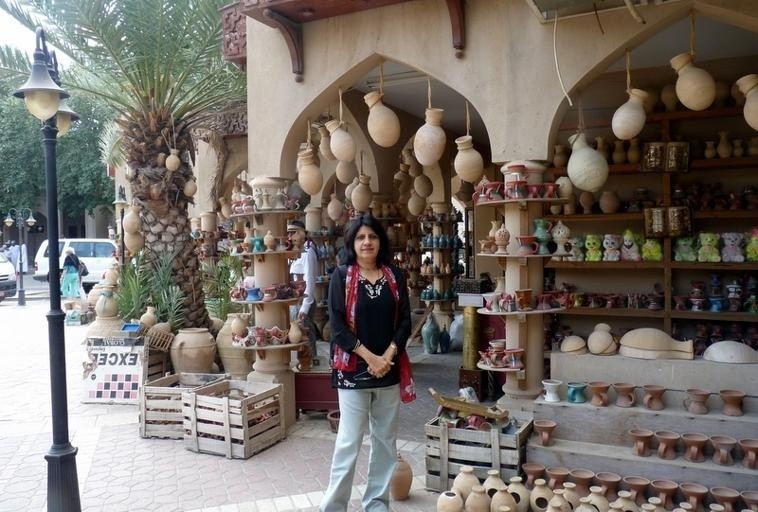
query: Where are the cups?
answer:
[227,239,313,303]
[470,168,562,375]
[520,373,758,512]
[562,280,755,314]
[430,409,517,442]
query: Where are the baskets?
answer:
[137,322,175,352]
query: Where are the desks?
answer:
[295,359,338,421]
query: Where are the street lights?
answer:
[11,27,94,512]
[109,183,130,270]
[5,205,37,307]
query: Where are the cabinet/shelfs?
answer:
[544,157,757,358]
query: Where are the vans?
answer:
[0,251,17,303]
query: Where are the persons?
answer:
[321,215,412,512]
[0,240,28,274]
[59,247,79,300]
[286,220,318,369]
[76,277,86,301]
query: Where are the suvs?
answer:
[28,235,130,291]
